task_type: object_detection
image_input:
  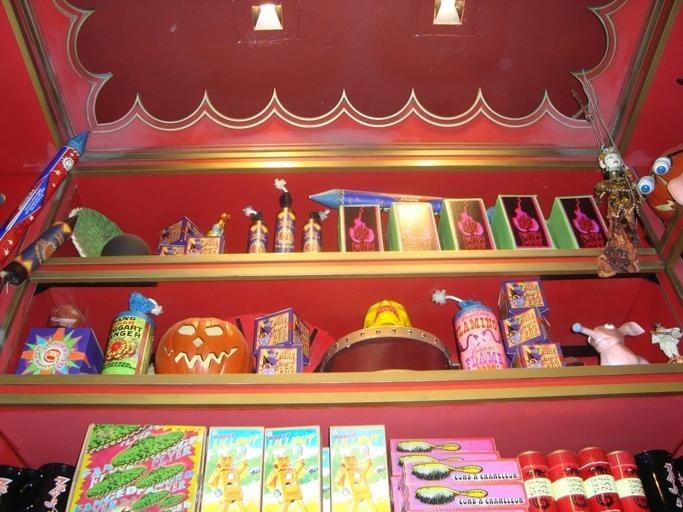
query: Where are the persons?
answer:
[648,322,682,363]
[592,145,643,278]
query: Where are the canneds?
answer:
[517,448,683,512]
[0,463,75,512]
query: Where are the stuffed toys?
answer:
[572,318,651,365]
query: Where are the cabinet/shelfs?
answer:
[607,2,683,305]
[1,154,682,472]
[1,2,73,353]
